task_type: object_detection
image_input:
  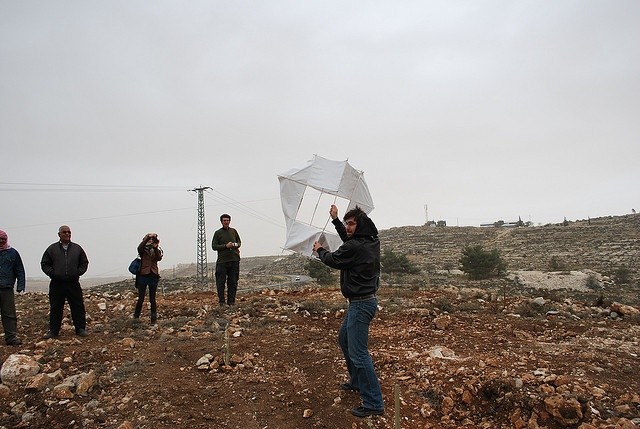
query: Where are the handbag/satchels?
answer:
[129,258,141,274]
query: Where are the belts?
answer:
[346,293,375,302]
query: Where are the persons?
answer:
[311,206,388,420]
[128,233,163,325]
[1,229,26,345]
[41,225,92,340]
[211,212,241,304]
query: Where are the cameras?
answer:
[151,236,159,245]
[230,243,238,250]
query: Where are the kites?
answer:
[274,154,375,264]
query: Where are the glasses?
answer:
[63,231,71,234]
[343,222,356,226]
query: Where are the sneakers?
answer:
[150,319,156,325]
[43,332,57,338]
[7,337,21,344]
[351,406,384,417]
[77,329,87,335]
[341,384,351,389]
